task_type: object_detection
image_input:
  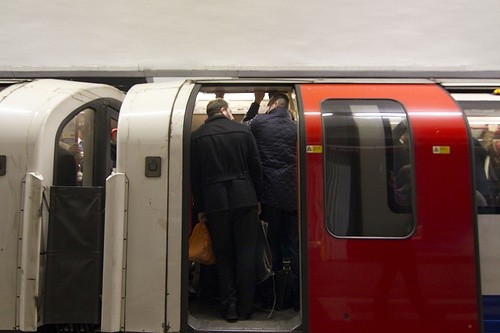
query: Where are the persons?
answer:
[241,90,300,311]
[189,99,263,322]
[54,128,116,186]
[200,92,500,299]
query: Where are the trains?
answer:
[0,0,500,332]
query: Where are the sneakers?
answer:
[237,302,255,321]
[221,299,236,322]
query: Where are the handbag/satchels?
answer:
[273,242,299,311]
